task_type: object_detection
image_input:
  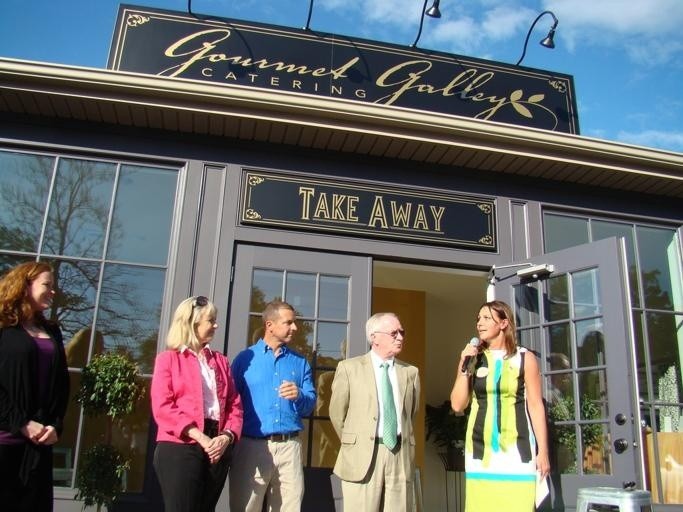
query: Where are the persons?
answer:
[62,325,105,449]
[250,328,269,345]
[1,260,72,510]
[329,312,421,512]
[449,300,552,512]
[229,301,316,512]
[544,353,576,473]
[316,338,346,468]
[151,294,245,512]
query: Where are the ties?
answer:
[381,363,398,451]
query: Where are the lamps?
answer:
[409,0,441,48]
[516,11,558,66]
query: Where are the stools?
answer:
[576,487,653,512]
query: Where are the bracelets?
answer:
[456,368,471,378]
[220,430,235,445]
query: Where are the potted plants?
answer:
[425,400,472,467]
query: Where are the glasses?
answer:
[379,330,405,336]
[196,294,209,305]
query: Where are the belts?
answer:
[375,436,400,444]
[263,433,298,440]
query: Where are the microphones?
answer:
[460,334,480,375]
